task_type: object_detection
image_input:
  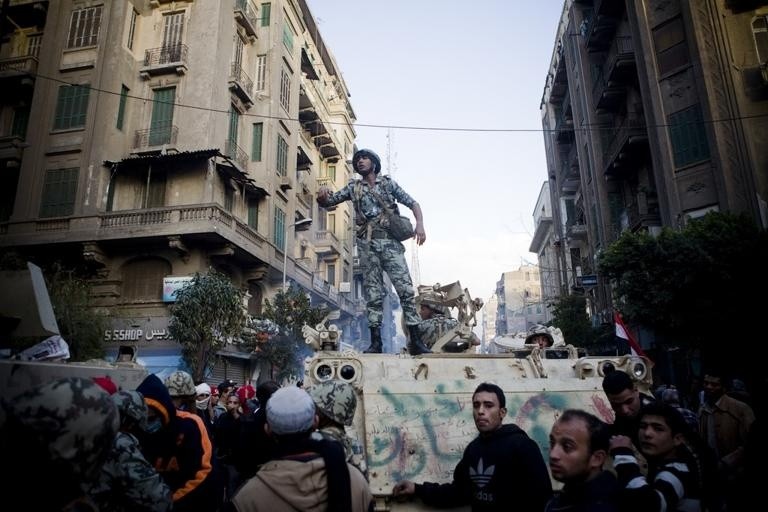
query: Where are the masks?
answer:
[145,417,162,435]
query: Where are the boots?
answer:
[363,327,383,353]
[406,326,434,355]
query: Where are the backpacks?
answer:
[0,374,120,512]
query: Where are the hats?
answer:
[524,324,554,346]
[197,371,237,397]
[265,386,315,434]
[312,380,356,426]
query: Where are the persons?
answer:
[314,147,436,355]
[0,348,768,512]
[413,289,480,352]
[523,326,555,358]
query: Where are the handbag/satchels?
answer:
[390,215,414,240]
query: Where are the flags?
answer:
[611,310,650,368]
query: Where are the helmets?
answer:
[164,370,196,396]
[111,389,149,433]
[352,149,381,174]
[420,292,447,314]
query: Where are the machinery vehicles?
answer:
[301,281,655,510]
[0,262,152,409]
